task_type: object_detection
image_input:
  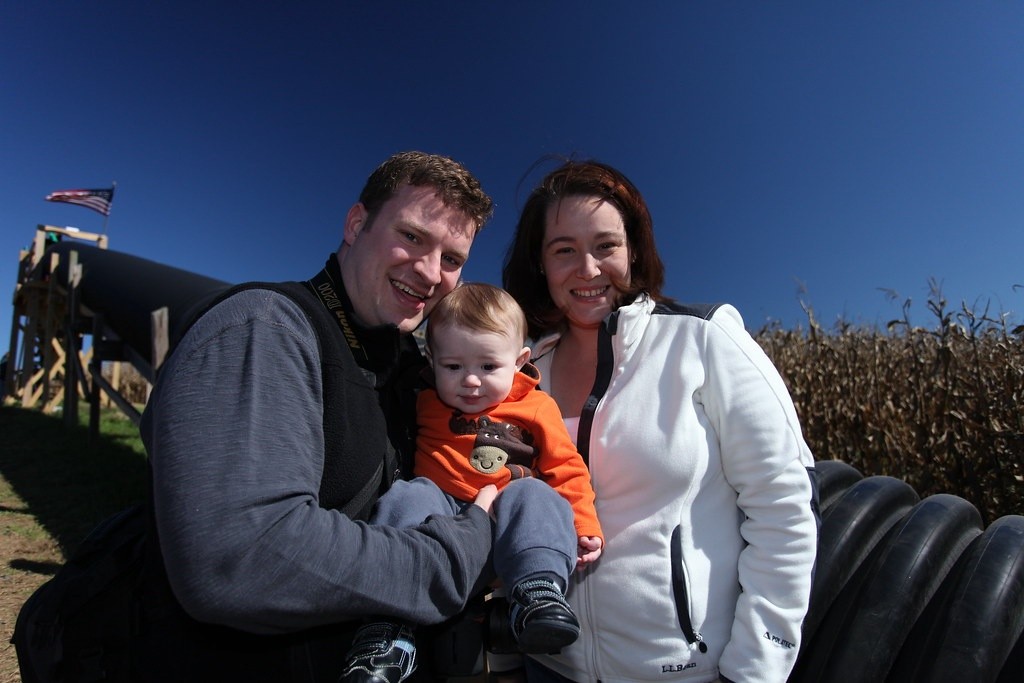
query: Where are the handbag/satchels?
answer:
[10,504,145,683]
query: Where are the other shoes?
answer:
[509,577,579,655]
[338,615,427,683]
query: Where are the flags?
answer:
[45,187,113,216]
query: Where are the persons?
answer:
[138,149,823,683]
